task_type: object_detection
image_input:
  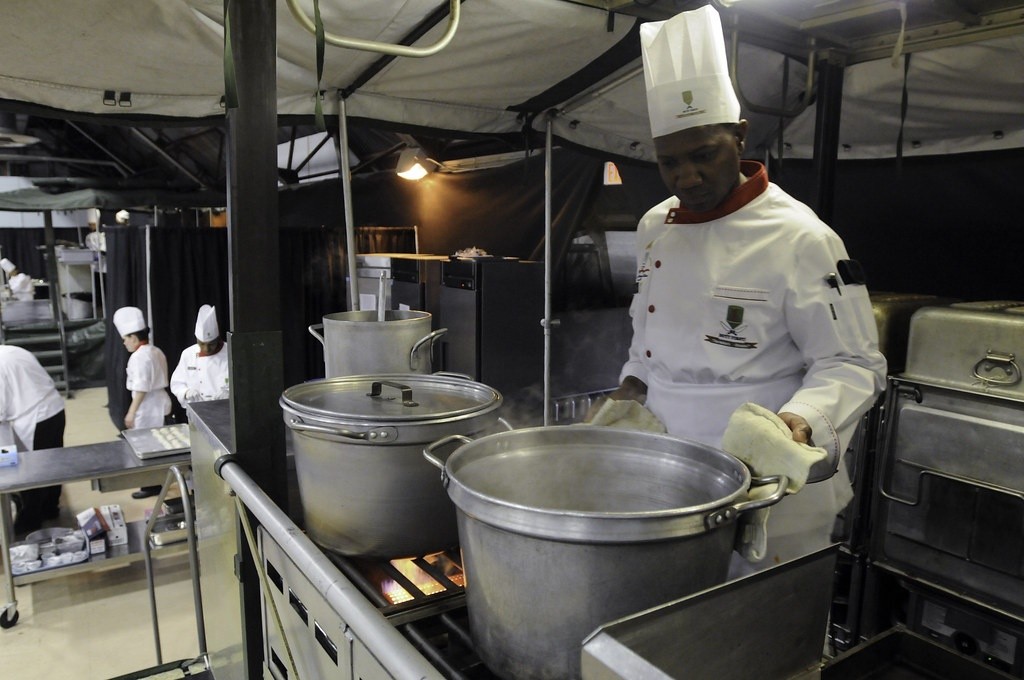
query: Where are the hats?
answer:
[112,306,147,339]
[0,258,16,274]
[86,208,100,224]
[195,304,220,343]
[638,3,742,138]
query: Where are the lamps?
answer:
[395,147,437,182]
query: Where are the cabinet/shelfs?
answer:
[0,321,71,399]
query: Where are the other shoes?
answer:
[132,485,162,499]
[14,505,60,534]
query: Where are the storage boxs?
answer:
[101,504,129,547]
[76,507,111,557]
[60,249,94,261]
[1,299,54,321]
[144,507,165,520]
[162,496,195,514]
[898,301,1024,403]
[0,445,18,467]
[148,515,198,547]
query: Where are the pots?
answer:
[281,375,502,557]
[309,311,448,379]
[423,425,789,680]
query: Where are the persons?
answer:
[112,306,171,499]
[584,4,887,584]
[0,258,36,300]
[0,344,66,541]
[169,303,230,410]
[115,209,130,226]
[86,207,107,274]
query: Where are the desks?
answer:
[0,439,198,626]
